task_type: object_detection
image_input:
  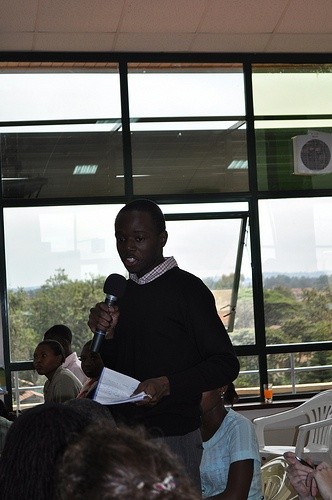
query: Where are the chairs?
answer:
[253,389,332,500]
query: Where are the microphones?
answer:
[89,274,127,354]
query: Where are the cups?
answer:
[263,384,273,404]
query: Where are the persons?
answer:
[0,325,202,500]
[89,203,240,496]
[198,384,262,500]
[283,451,332,500]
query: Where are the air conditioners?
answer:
[290,131,332,175]
